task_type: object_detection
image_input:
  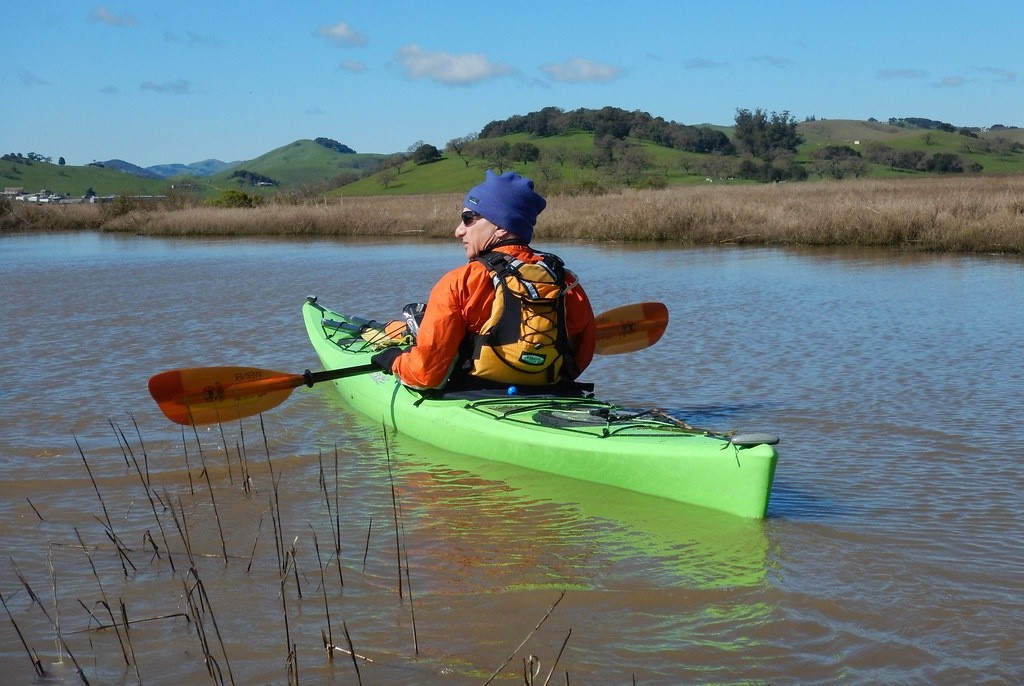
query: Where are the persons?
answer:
[371,171,597,391]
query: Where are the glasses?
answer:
[460,210,482,226]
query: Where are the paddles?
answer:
[147,301,670,427]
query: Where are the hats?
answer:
[463,169,547,242]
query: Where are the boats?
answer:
[302,294,779,521]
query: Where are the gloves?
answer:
[370,347,404,374]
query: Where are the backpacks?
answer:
[467,251,574,393]
